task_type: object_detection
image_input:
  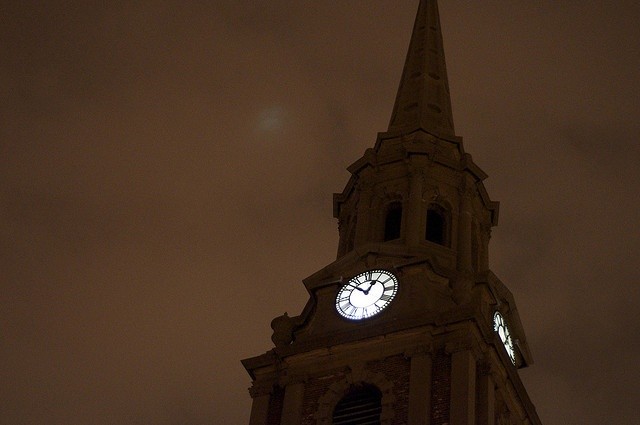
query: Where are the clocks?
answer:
[334,269,399,322]
[492,311,516,368]
